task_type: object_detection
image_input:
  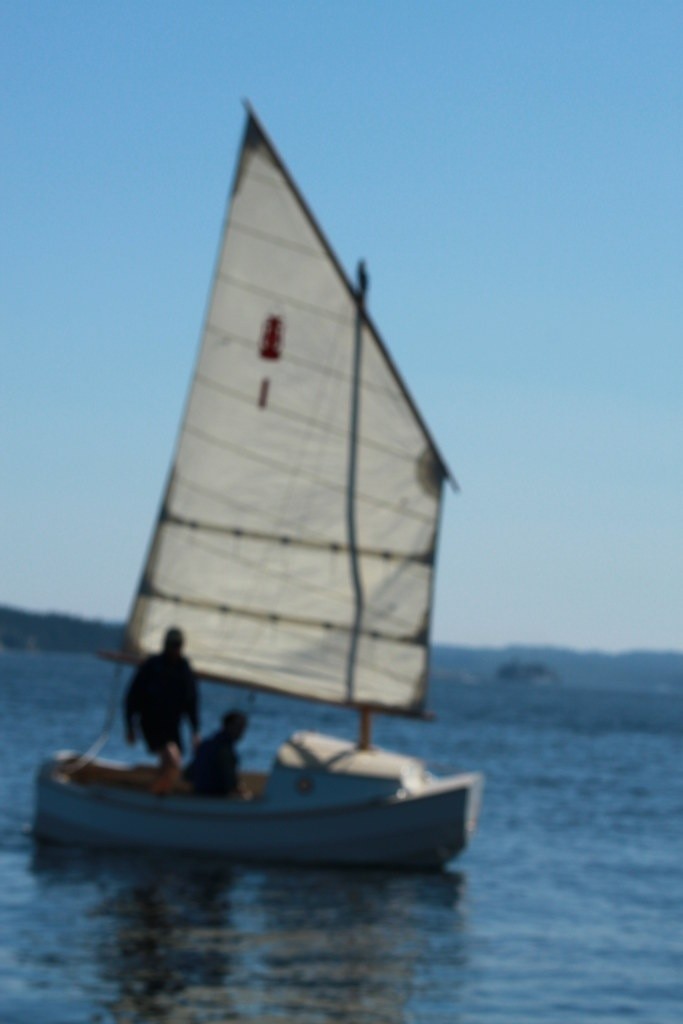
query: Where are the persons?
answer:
[121,629,202,770]
[182,708,247,802]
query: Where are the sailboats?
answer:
[30,84,480,871]
